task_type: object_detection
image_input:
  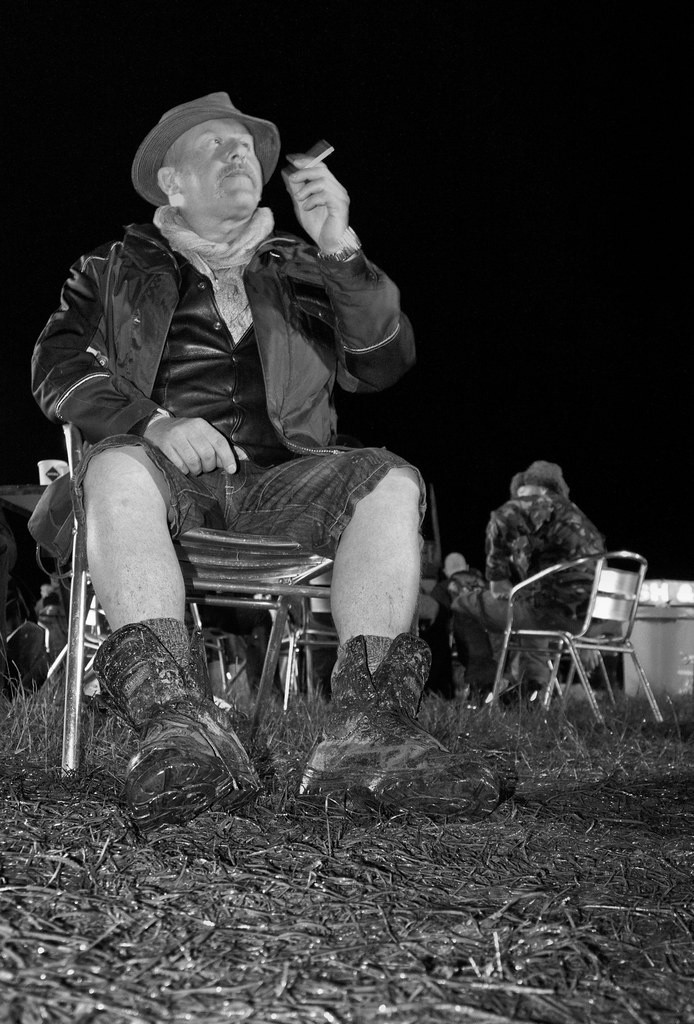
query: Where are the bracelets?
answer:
[317,226,362,262]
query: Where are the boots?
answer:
[298,631,501,826]
[90,618,265,833]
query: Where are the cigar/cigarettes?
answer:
[301,145,335,169]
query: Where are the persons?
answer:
[33,584,68,662]
[29,90,503,834]
[449,459,607,715]
[442,548,487,597]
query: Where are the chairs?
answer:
[49,415,331,775]
[485,551,666,731]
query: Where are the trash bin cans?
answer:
[626,580,694,710]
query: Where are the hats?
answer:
[130,92,281,207]
[510,460,570,499]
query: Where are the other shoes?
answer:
[525,679,558,713]
[467,676,510,712]
